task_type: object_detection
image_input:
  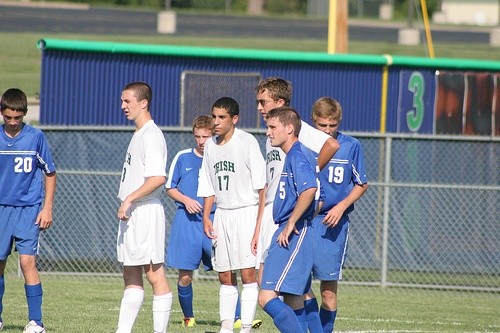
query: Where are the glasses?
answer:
[256,99,277,106]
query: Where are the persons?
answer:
[310,95,367,333]
[115,82,173,333]
[166,114,262,330]
[0,87,57,333]
[195,96,267,333]
[257,107,327,333]
[255,76,340,333]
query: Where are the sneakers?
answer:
[21,320,45,333]
[233,318,262,331]
[0,322,4,331]
[181,316,195,327]
